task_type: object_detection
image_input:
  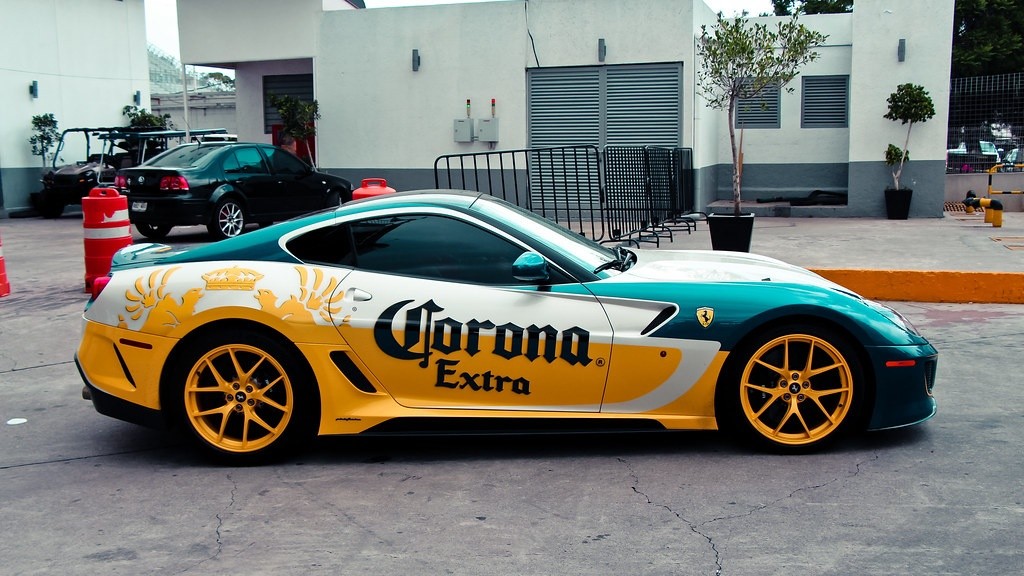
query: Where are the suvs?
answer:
[38,125,237,218]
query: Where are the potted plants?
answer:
[883,83,936,220]
[29,113,64,206]
[696,5,830,254]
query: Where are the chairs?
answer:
[88,154,137,170]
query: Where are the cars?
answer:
[71,190,939,465]
[946,141,1024,173]
[115,140,356,239]
